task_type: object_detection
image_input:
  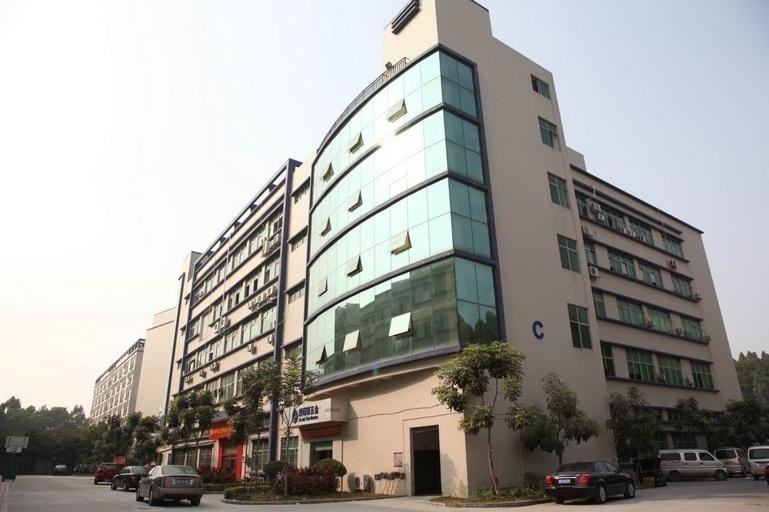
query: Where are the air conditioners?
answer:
[248,284,278,310]
[352,473,370,491]
[581,224,593,237]
[183,361,218,383]
[589,265,601,279]
[247,334,275,353]
[213,317,229,333]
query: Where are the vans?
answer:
[657,446,769,481]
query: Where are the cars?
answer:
[544,461,636,505]
[51,464,72,476]
[94,463,204,507]
